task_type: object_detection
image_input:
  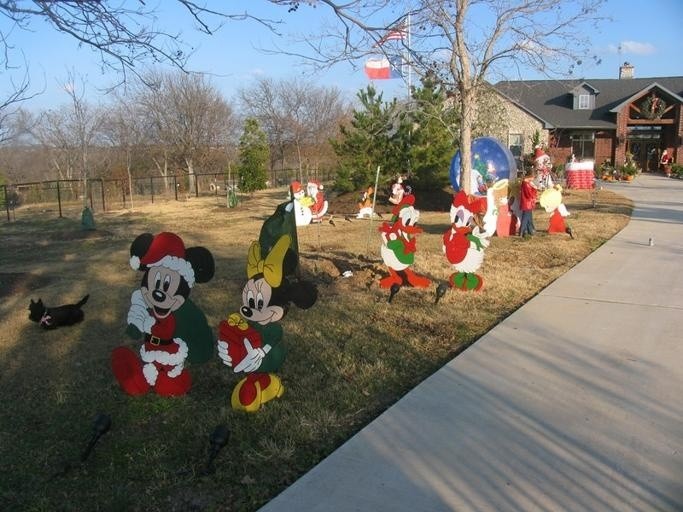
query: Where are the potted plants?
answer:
[596,164,638,182]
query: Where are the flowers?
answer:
[640,97,666,120]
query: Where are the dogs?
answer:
[28,293,90,330]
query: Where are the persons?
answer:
[659,149,668,170]
[519,169,537,237]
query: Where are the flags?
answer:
[365,18,408,80]
